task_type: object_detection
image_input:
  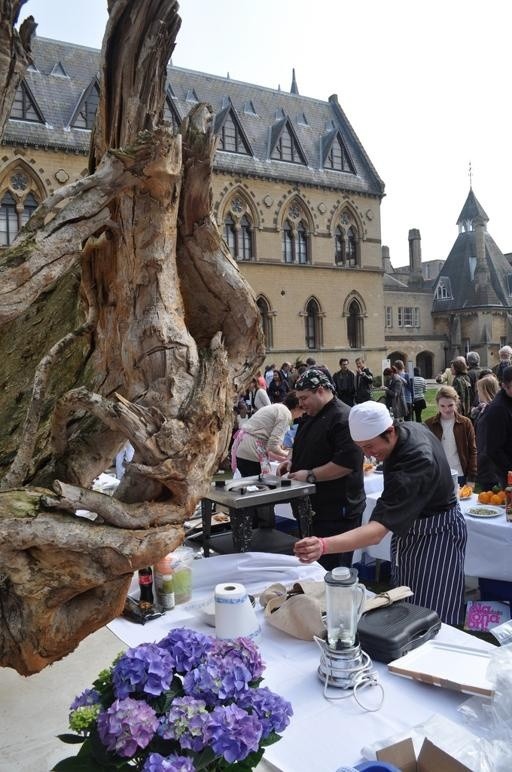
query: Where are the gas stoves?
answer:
[198,471,319,554]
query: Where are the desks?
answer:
[0,551,512,772]
[235,461,458,567]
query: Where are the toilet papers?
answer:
[214,581,262,646]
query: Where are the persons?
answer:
[288,368,367,572]
[292,400,468,627]
[229,357,426,534]
[424,345,512,492]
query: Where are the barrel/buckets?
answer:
[153,547,194,611]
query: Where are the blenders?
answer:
[316,566,368,688]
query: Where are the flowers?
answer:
[51,629,293,772]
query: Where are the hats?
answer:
[347,399,395,442]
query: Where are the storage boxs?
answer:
[377,737,473,772]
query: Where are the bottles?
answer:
[503,470,512,522]
[158,575,175,610]
[137,564,154,604]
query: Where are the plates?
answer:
[375,467,385,474]
[464,504,503,518]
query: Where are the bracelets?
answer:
[320,538,326,556]
